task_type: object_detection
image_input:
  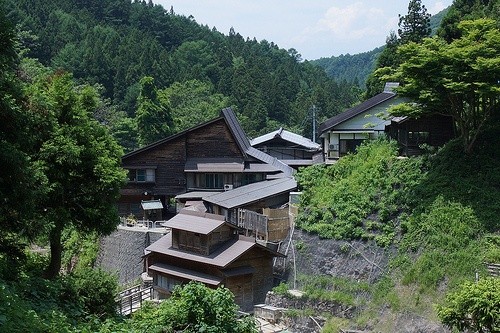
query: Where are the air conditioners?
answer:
[329,144,337,150]
[224,184,233,190]
